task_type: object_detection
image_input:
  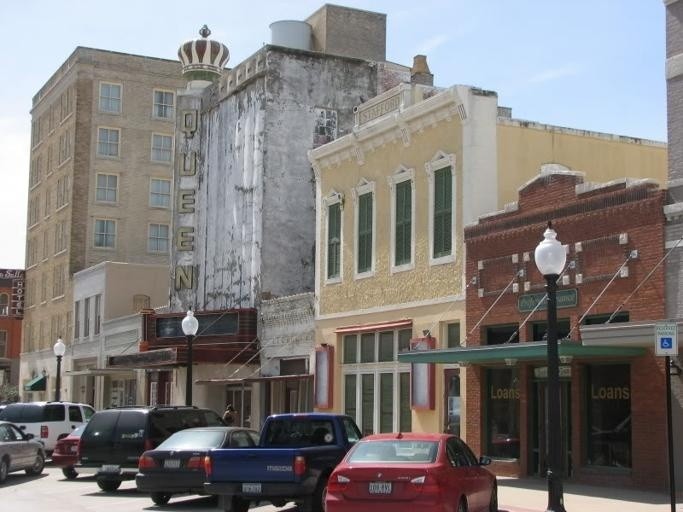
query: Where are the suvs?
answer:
[72,404,240,491]
[0,401,96,459]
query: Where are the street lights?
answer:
[53,335,65,402]
[534,228,567,512]
[181,306,199,405]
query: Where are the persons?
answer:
[219,404,240,428]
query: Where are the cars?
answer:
[136,427,261,505]
[0,421,46,484]
[326,431,498,512]
[51,425,85,479]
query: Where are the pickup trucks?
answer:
[203,412,365,512]
